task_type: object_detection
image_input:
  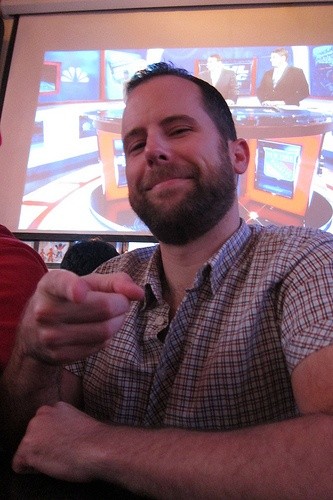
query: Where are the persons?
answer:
[256,48,309,107]
[1,59,332,499]
[196,53,237,107]
[58,239,120,279]
[0,224,50,365]
